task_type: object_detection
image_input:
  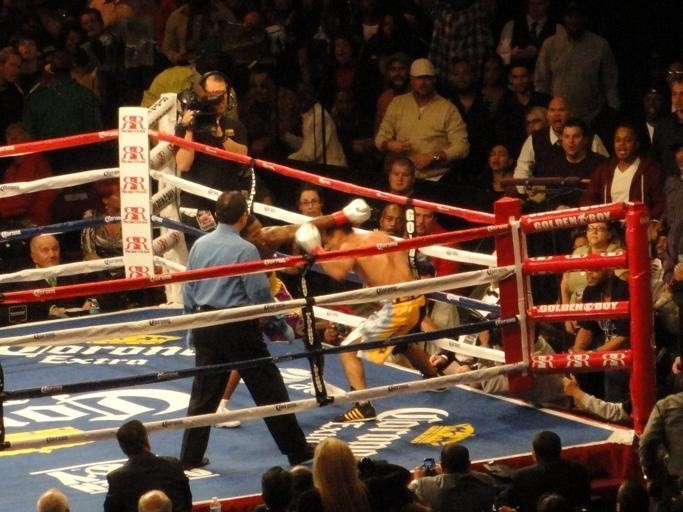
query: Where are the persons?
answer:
[2,0,682,512]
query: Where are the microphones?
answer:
[227,104,233,111]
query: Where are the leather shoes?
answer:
[181,458,208,470]
[289,443,317,466]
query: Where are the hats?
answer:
[410,58,436,77]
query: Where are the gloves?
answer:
[332,198,371,227]
[295,222,324,255]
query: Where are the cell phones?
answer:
[424,458,435,472]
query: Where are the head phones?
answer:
[205,72,238,113]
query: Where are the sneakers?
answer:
[335,402,376,422]
[215,409,240,427]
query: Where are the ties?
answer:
[48,277,55,286]
